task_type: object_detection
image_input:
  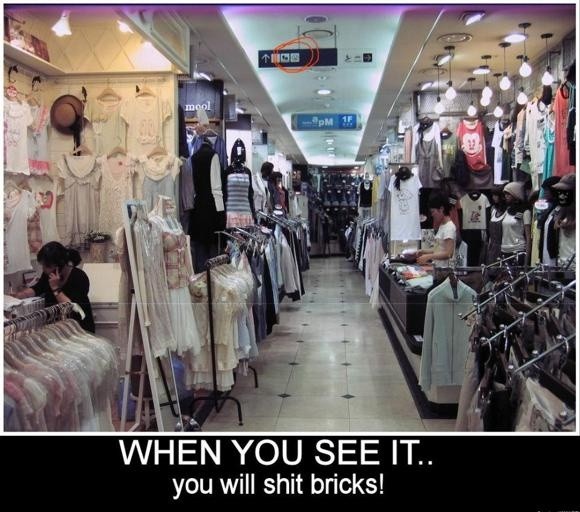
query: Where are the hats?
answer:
[552,174,575,191]
[50,95,85,135]
[492,182,525,201]
[541,176,561,190]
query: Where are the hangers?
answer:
[96,80,155,102]
[448,249,576,431]
[202,123,219,137]
[7,66,43,107]
[4,175,21,193]
[469,190,481,197]
[68,137,168,158]
[195,254,238,285]
[4,301,83,370]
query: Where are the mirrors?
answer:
[118,196,185,432]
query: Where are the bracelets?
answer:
[53,287,63,295]
[22,290,30,298]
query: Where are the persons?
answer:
[486,173,575,272]
[191,128,225,272]
[8,240,95,334]
[415,191,457,281]
[224,137,258,229]
[147,194,208,431]
[116,199,154,411]
[252,161,274,213]
[270,171,289,214]
